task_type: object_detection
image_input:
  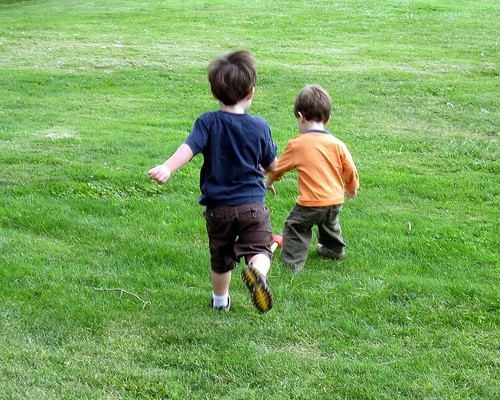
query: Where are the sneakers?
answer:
[317,244,345,259]
[242,265,273,314]
[212,292,231,314]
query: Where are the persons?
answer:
[263,84,361,276]
[147,50,274,312]
